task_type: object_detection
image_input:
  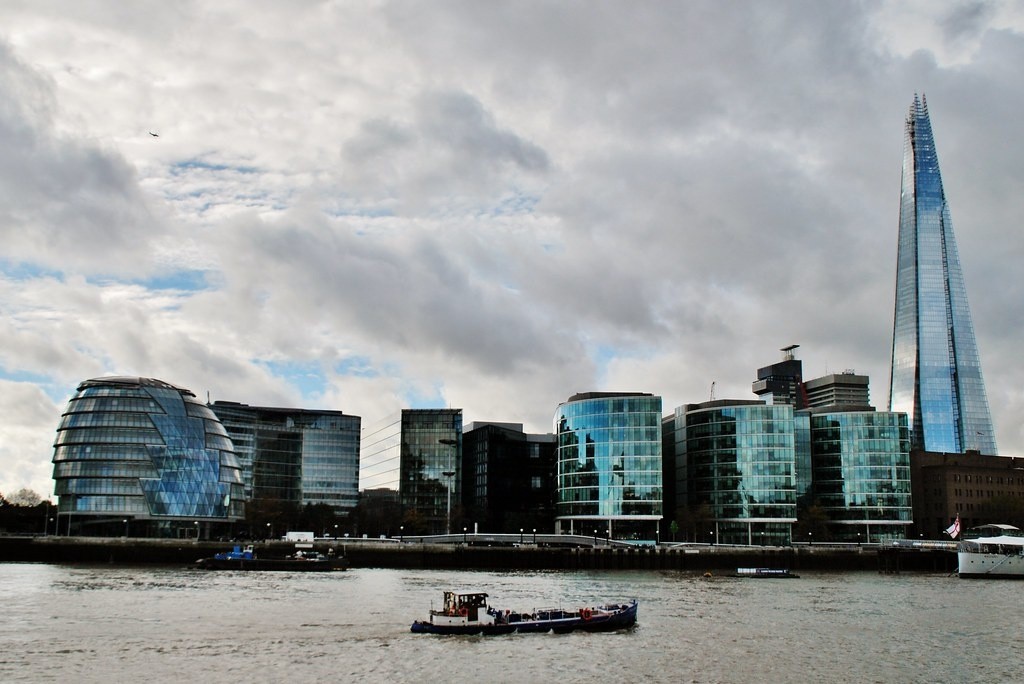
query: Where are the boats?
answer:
[410,591,638,635]
[734,567,801,578]
[957,525,1024,578]
[197,544,347,572]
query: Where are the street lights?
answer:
[809,532,811,546]
[761,532,764,546]
[594,530,597,545]
[656,530,659,545]
[533,529,536,544]
[400,526,403,542]
[335,525,338,541]
[521,529,523,544]
[606,530,608,545]
[464,528,467,543]
[857,533,861,547]
[710,531,713,546]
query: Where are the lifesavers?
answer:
[458,607,469,617]
[581,609,593,620]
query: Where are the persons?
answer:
[966,545,971,553]
[456,599,464,607]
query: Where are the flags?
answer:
[945,516,961,541]
